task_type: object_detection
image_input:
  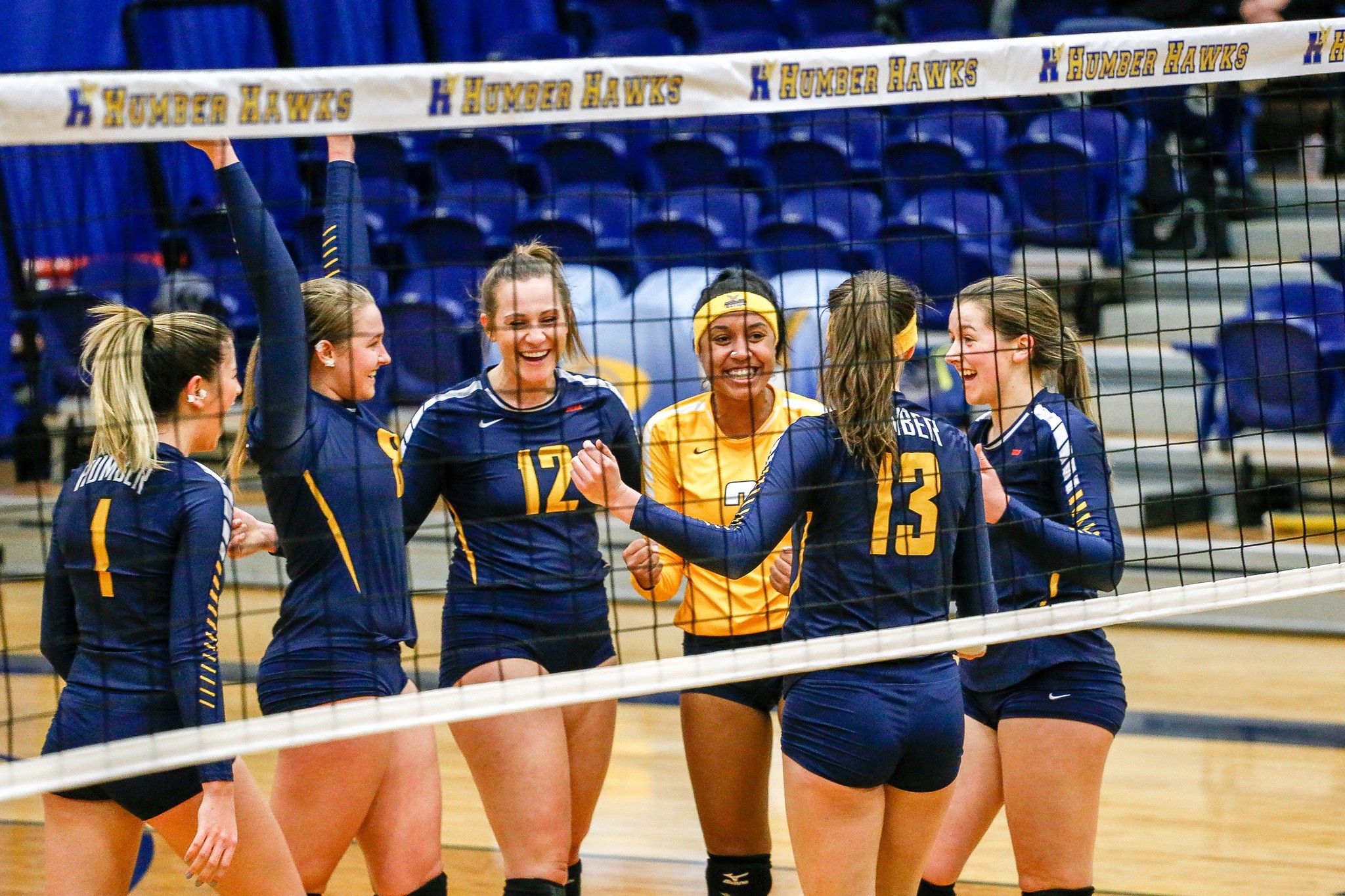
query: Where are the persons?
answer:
[564,269,1000,896]
[1156,0,1345,180]
[908,274,1127,896]
[623,269,833,896]
[224,240,642,896]
[34,303,309,896]
[182,135,447,896]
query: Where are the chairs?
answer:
[0,20,1344,461]
